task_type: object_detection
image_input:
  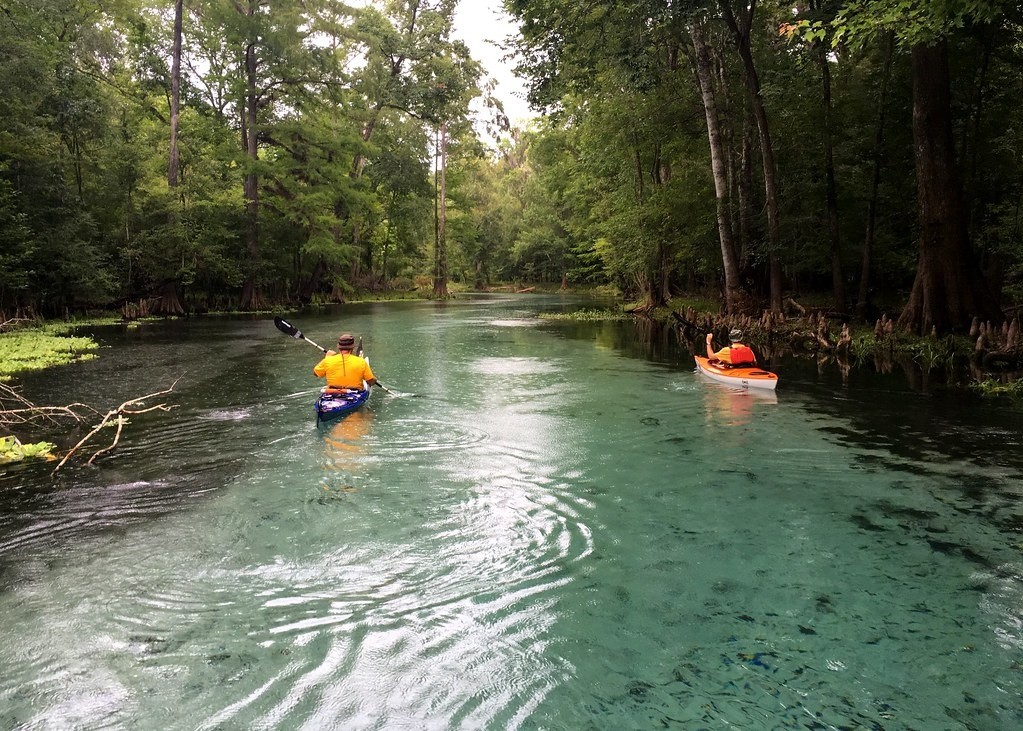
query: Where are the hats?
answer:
[728,329,744,343]
[336,334,355,350]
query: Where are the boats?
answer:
[694,355,779,390]
[313,355,372,421]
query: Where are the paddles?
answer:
[672,311,725,346]
[274,316,395,396]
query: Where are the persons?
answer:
[706,329,758,368]
[312,333,378,393]
